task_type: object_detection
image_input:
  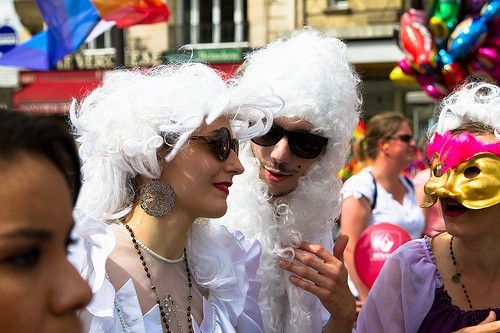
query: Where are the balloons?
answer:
[353,223,410,290]
[388,0,500,99]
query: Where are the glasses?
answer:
[187,127,239,161]
[248,118,328,159]
[389,134,413,142]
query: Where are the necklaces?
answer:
[449,236,486,326]
[120,215,195,333]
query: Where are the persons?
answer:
[354,76,500,333]
[202,24,361,333]
[0,101,95,333]
[334,110,426,321]
[69,56,270,333]
